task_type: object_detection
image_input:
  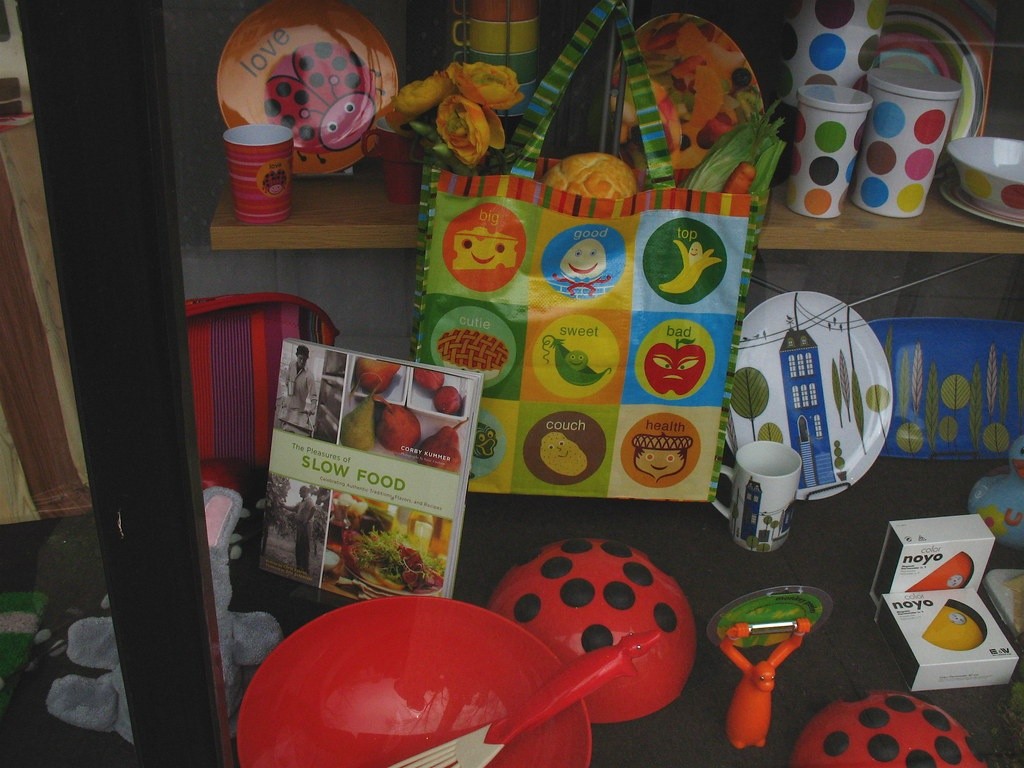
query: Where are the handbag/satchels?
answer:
[410,0,769,503]
[184,290,340,468]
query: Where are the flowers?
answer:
[388,60,526,172]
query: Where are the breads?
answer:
[538,152,637,201]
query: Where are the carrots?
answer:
[722,162,756,194]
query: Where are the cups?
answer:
[728,439,802,555]
[450,0,540,144]
[771,0,964,219]
[361,115,423,205]
[224,123,296,225]
[414,520,433,559]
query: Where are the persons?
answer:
[285,344,317,415]
[276,485,315,574]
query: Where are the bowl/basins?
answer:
[322,544,346,583]
[949,136,1024,215]
[235,594,592,768]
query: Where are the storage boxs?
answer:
[869,514,996,609]
[874,588,1020,692]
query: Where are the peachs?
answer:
[414,368,445,392]
[434,386,464,415]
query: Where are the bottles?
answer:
[320,498,353,566]
[406,510,436,560]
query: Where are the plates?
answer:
[610,13,765,171]
[344,566,445,596]
[218,1,400,177]
[724,290,895,502]
[940,171,1024,230]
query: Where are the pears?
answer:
[339,358,472,472]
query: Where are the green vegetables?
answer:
[351,524,446,586]
[682,91,786,194]
